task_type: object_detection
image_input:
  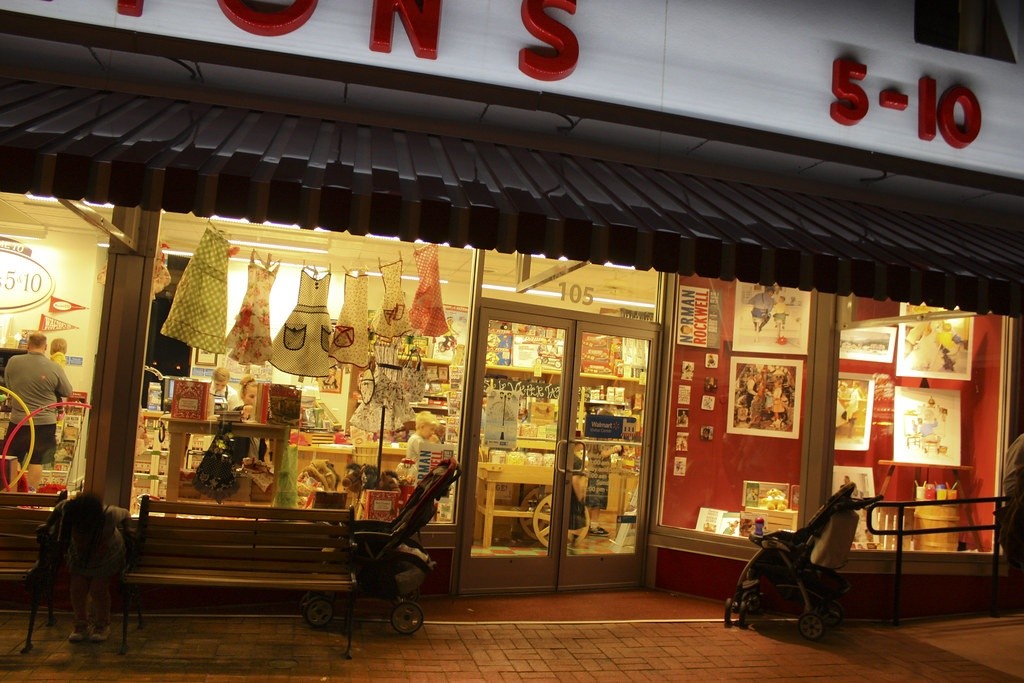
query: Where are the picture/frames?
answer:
[316,364,343,393]
[726,356,803,440]
[195,348,217,366]
[834,372,875,452]
[831,466,880,545]
[896,301,975,381]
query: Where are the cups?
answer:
[937,489,946,500]
[947,490,957,499]
[925,484,935,499]
[916,486,925,499]
[936,484,945,490]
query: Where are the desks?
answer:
[879,460,984,552]
[160,412,291,518]
[745,506,798,533]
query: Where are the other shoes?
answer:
[90,626,110,641]
[590,527,609,536]
[70,625,90,642]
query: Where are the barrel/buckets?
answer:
[914,504,959,552]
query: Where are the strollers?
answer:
[299,457,462,632]
[724,481,884,638]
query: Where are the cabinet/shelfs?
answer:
[576,400,629,423]
[357,351,452,410]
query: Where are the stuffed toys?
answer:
[374,471,401,496]
[360,464,380,490]
[342,463,361,513]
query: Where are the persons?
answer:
[49,338,67,421]
[585,410,622,536]
[747,287,774,332]
[406,411,439,459]
[746,374,762,423]
[36,493,136,641]
[770,381,782,428]
[773,296,785,329]
[841,381,864,426]
[993,433,1024,573]
[0,332,72,471]
[233,377,258,420]
[203,367,238,411]
[913,418,939,453]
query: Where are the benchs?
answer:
[0,488,67,655]
[115,495,358,661]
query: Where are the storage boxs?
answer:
[364,490,400,523]
[171,380,211,420]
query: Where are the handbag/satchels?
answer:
[193,425,240,500]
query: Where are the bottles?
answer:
[396,457,417,509]
[306,408,324,428]
[755,517,764,535]
[147,382,162,411]
